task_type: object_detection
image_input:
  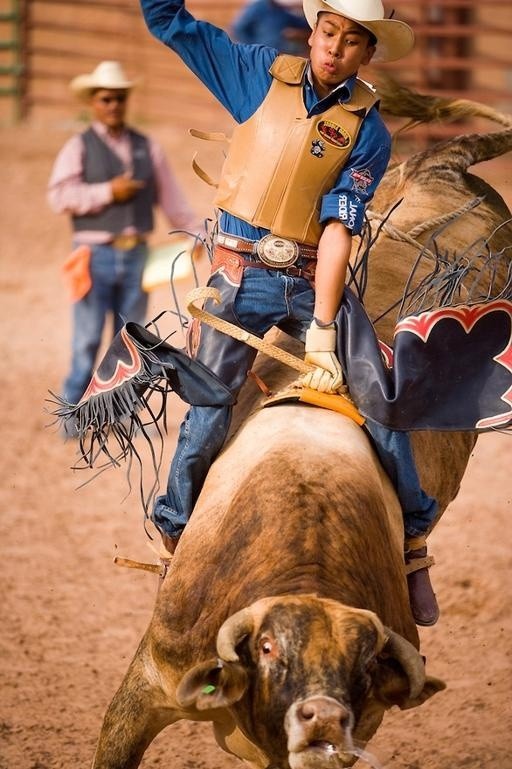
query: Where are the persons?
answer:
[233,0,311,54]
[45,61,208,440]
[139,1,440,627]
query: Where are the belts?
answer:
[217,227,317,267]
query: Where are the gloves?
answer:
[301,317,344,394]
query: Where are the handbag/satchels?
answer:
[62,244,93,303]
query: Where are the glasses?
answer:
[102,95,126,104]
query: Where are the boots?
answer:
[157,532,180,585]
[402,533,440,626]
[302,0,415,64]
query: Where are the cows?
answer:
[91,75,512,769]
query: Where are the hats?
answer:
[70,60,136,96]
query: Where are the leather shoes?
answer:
[118,417,133,434]
[56,418,77,437]
